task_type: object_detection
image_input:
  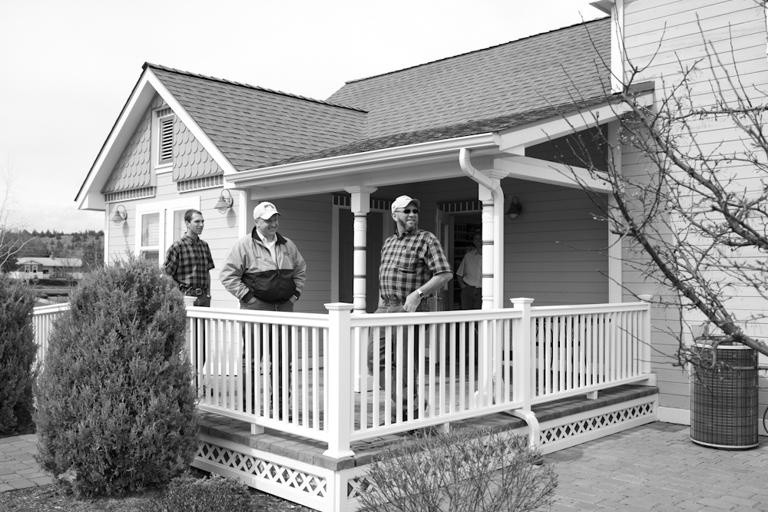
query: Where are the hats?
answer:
[391,195,421,214]
[253,201,281,221]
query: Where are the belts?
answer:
[184,287,207,297]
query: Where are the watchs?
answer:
[418,288,425,301]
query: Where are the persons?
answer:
[164,208,214,397]
[220,200,308,420]
[456,232,481,365]
[369,193,454,435]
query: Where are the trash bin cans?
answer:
[690,336,760,449]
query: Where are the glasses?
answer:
[395,209,418,214]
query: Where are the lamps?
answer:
[505,195,522,221]
[213,188,233,215]
[111,204,127,226]
